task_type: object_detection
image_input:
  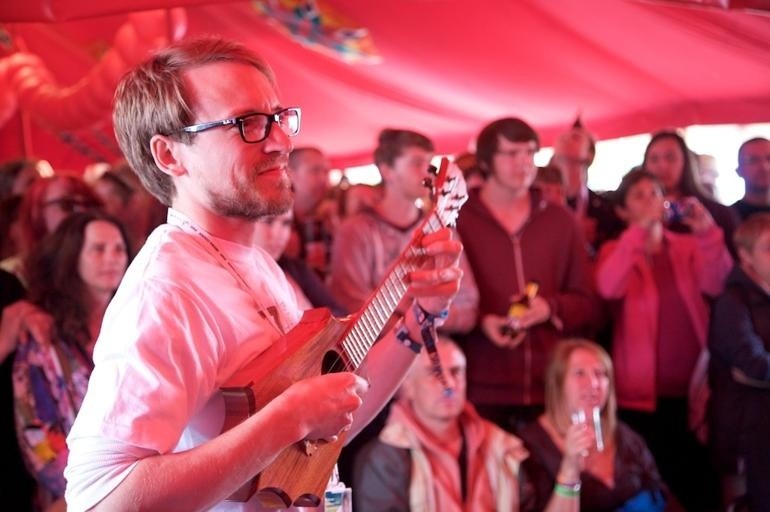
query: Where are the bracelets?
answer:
[408,298,450,354]
[394,323,421,355]
[555,481,581,497]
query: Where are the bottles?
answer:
[504,278,540,339]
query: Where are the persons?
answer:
[593,170,734,511]
[255,187,348,318]
[453,118,600,428]
[706,215,770,510]
[729,137,770,215]
[643,133,735,252]
[518,338,677,512]
[64,34,465,512]
[0,162,54,263]
[328,129,480,338]
[554,121,603,221]
[85,165,167,259]
[14,213,130,509]
[0,178,109,511]
[282,147,381,283]
[351,339,529,511]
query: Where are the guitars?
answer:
[220,155,469,511]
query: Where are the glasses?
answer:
[497,146,536,160]
[41,193,100,213]
[162,105,302,143]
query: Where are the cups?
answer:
[570,406,605,458]
